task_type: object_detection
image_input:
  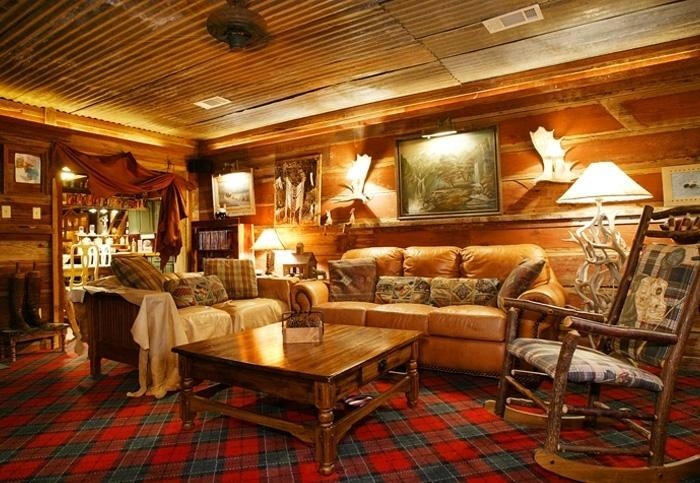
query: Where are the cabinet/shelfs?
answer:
[61,174,175,288]
[192,219,254,273]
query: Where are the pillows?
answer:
[432,277,499,308]
[375,274,432,305]
[165,278,197,308]
[181,275,228,305]
[328,259,375,303]
[110,254,167,291]
[203,257,258,299]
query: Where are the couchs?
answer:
[82,272,299,396]
[289,244,565,392]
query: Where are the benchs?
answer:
[0,323,69,362]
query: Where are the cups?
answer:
[89,224,95,236]
[79,226,84,235]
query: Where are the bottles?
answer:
[132,238,137,253]
[62,181,143,208]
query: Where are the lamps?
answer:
[249,229,285,277]
[555,160,653,350]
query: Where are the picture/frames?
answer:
[394,123,504,221]
[13,151,41,184]
[662,166,700,208]
[212,164,255,218]
[273,154,322,225]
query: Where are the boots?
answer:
[8,275,47,331]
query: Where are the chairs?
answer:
[483,205,700,482]
